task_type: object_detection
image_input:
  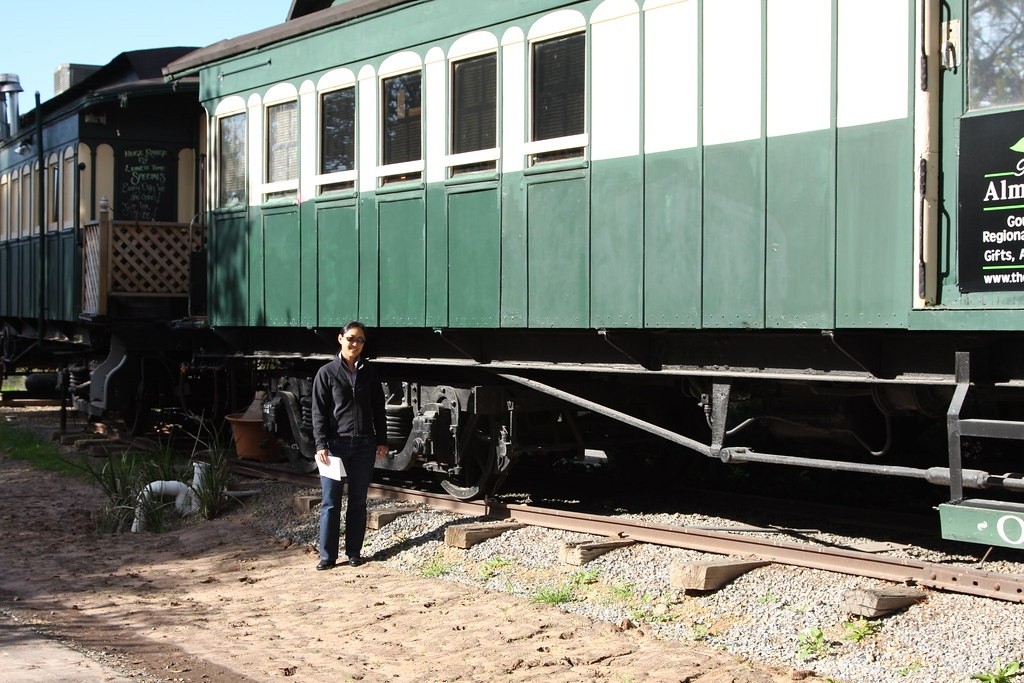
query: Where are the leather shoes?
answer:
[316,559,335,570]
[349,554,360,566]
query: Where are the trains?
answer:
[0,1,1023,548]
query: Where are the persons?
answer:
[312,321,388,570]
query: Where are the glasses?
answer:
[342,335,365,344]
